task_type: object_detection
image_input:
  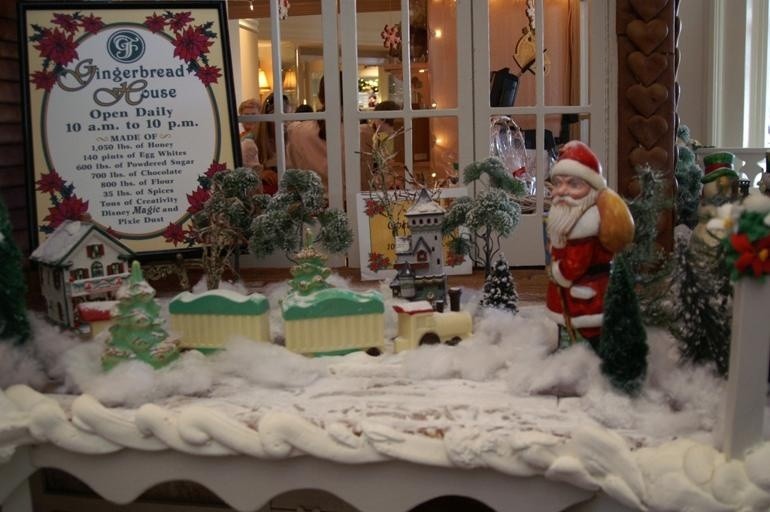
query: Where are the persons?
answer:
[239,71,400,211]
[543,138,636,348]
